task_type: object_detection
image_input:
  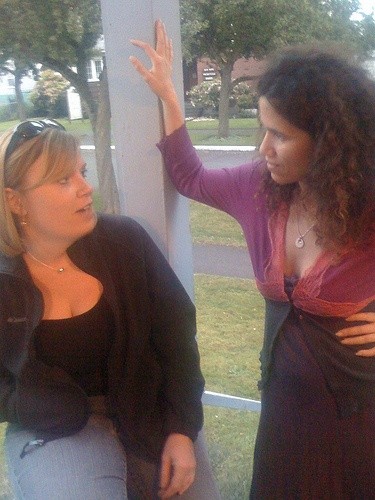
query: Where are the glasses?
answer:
[4,119,66,159]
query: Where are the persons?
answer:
[130,19,375,500]
[0,122,217,500]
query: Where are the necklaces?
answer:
[295,216,315,247]
[27,250,68,272]
